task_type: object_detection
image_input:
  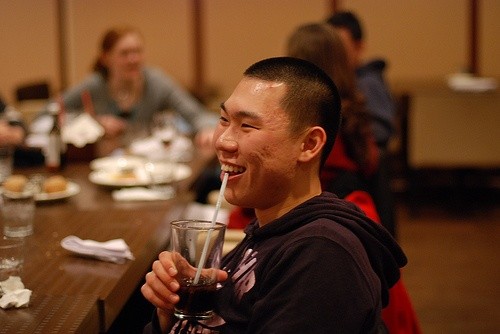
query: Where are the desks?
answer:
[0,150,217,334]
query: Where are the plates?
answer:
[34,177,81,202]
[88,154,194,187]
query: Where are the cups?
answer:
[0,239,26,285]
[171,219,227,321]
[0,186,35,237]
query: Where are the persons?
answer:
[142,11,408,334]
[57,23,222,150]
[0,94,25,145]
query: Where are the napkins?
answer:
[59,231,132,262]
[111,187,177,201]
